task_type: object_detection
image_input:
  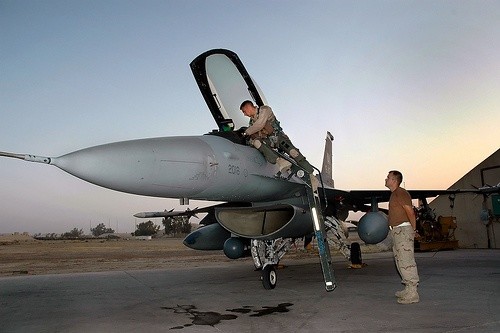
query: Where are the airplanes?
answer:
[0,49,499,291]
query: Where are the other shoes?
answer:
[395,285,409,298]
[276,157,292,173]
[309,174,319,191]
[397,285,420,304]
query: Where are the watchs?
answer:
[243,131,248,136]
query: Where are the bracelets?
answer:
[414,229,418,233]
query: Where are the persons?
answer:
[240,101,314,173]
[385,171,420,305]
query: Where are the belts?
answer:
[389,222,411,230]
